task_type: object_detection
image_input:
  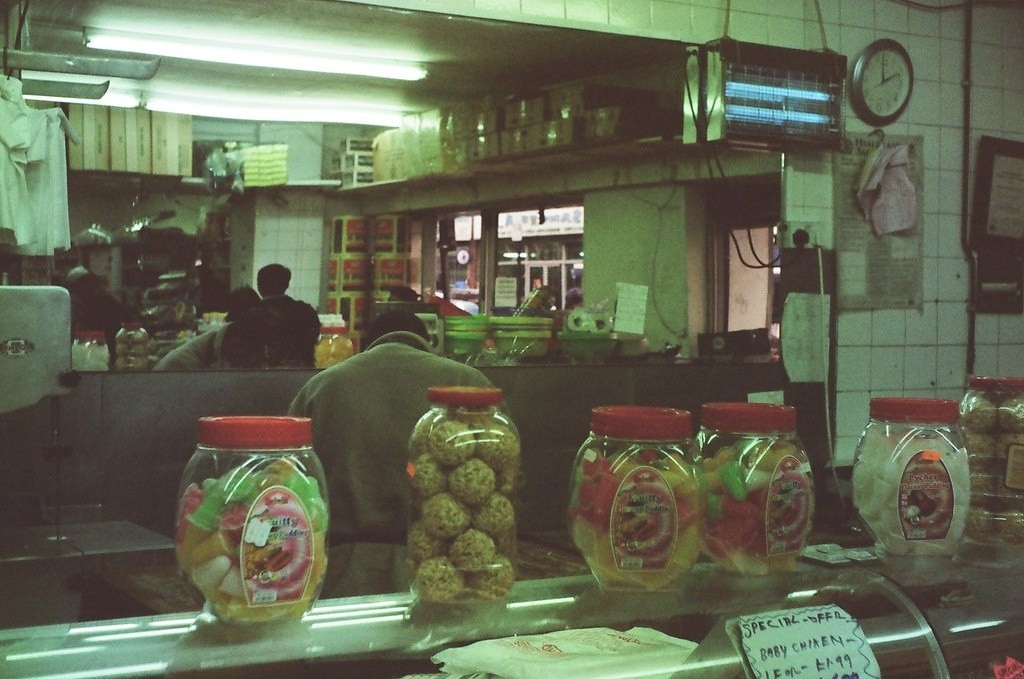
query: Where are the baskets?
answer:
[243,145,287,186]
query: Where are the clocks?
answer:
[851,38,916,127]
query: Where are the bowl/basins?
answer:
[558,330,618,355]
[488,317,553,356]
[444,314,489,355]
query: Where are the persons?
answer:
[387,285,417,311]
[157,263,323,371]
[287,306,511,601]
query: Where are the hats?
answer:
[361,308,430,348]
[222,286,261,321]
[257,264,291,296]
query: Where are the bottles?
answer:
[114,322,149,371]
[960,374,1024,540]
[178,415,328,627]
[696,403,812,574]
[71,330,110,371]
[853,398,973,555]
[313,327,355,369]
[404,384,518,604]
[572,406,704,592]
